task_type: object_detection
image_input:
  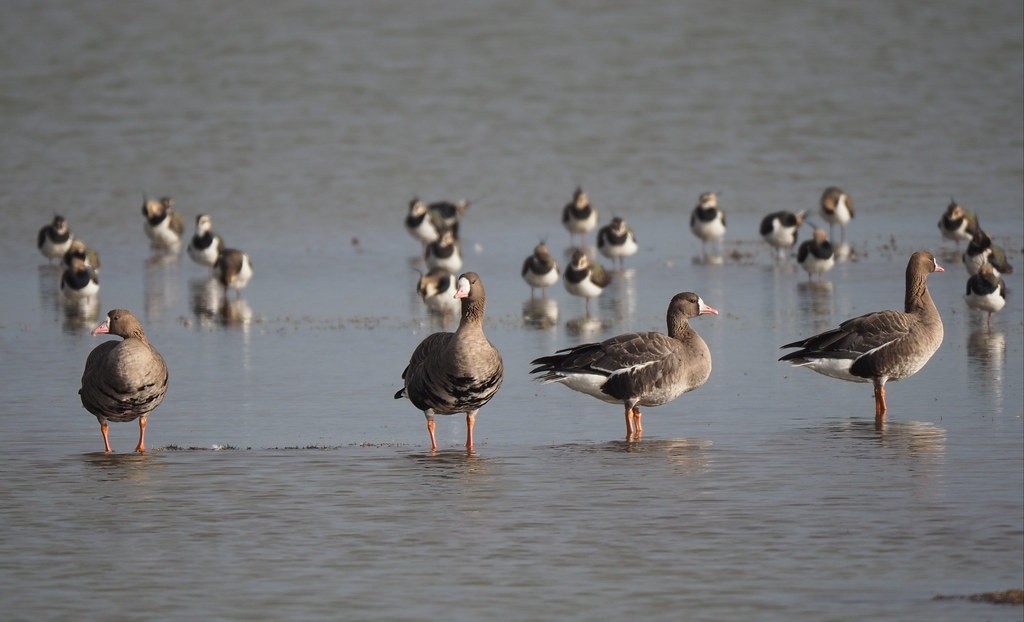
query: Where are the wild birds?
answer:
[529,290,719,440]
[79,309,168,454]
[36,186,1013,316]
[777,249,944,410]
[394,272,504,456]
